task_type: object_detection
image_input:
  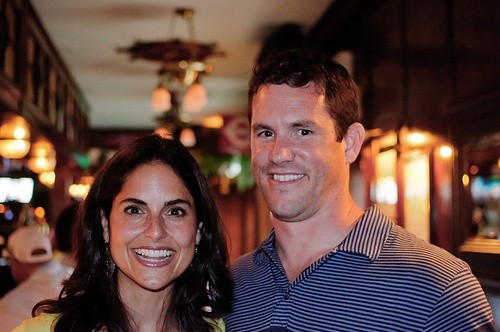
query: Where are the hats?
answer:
[5,226,53,262]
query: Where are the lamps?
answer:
[26,136,57,175]
[0,111,31,160]
[150,8,223,148]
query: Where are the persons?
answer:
[11,134,233,332]
[0,226,75,332]
[207,54,498,332]
